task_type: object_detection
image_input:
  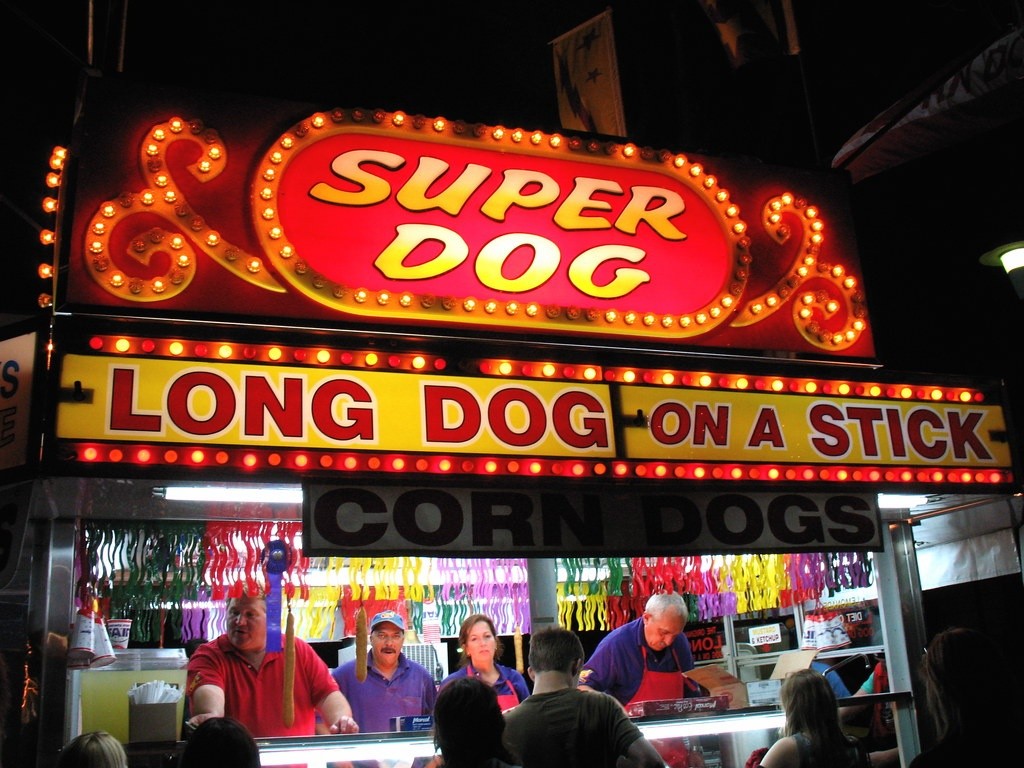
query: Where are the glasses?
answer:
[923,627,954,654]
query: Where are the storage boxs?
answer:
[685,665,747,711]
[129,682,179,744]
[629,696,729,718]
[389,715,433,732]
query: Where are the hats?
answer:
[371,610,405,635]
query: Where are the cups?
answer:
[104,618,132,649]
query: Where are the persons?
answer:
[180,717,262,768]
[434,677,523,768]
[59,731,128,768]
[577,590,700,713]
[757,661,872,768]
[838,653,900,768]
[502,628,665,768]
[440,615,530,713]
[186,579,360,738]
[909,628,1024,768]
[315,610,437,736]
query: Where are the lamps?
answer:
[151,486,304,504]
